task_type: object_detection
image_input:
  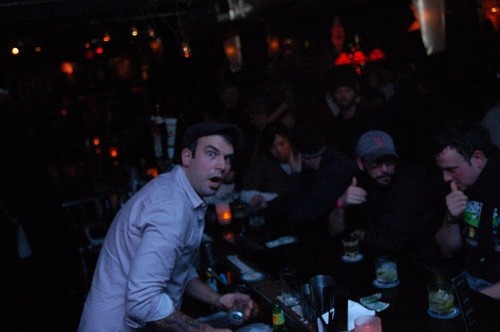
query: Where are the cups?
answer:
[374,255,398,285]
[216,202,232,224]
[343,238,359,258]
[354,315,382,332]
[427,280,455,315]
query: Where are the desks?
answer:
[202,172,500,332]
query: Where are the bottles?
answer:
[151,104,167,157]
[272,301,287,332]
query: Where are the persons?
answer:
[224,63,425,225]
[435,138,500,299]
[77,121,273,332]
[330,130,418,254]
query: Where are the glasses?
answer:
[364,154,395,169]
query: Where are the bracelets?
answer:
[336,197,343,209]
[444,210,460,224]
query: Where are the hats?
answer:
[182,121,240,147]
[355,131,399,162]
